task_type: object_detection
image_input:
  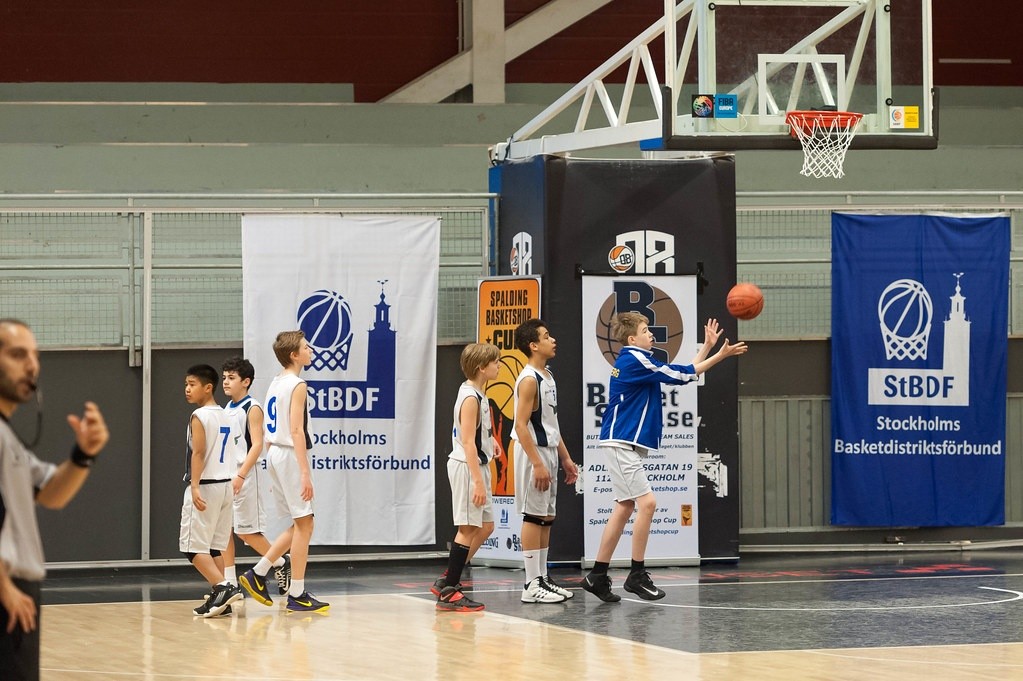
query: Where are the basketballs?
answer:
[726,282,765,320]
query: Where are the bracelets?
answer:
[70,440,96,468]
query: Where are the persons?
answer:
[184,331,330,617]
[509,319,578,604]
[0,316,110,681]
[581,313,749,603]
[430,342,502,612]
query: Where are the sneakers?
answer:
[238,568,273,606]
[431,578,485,612]
[286,590,330,612]
[521,575,574,603]
[623,572,666,600]
[192,582,246,618]
[579,574,621,603]
[273,554,292,596]
